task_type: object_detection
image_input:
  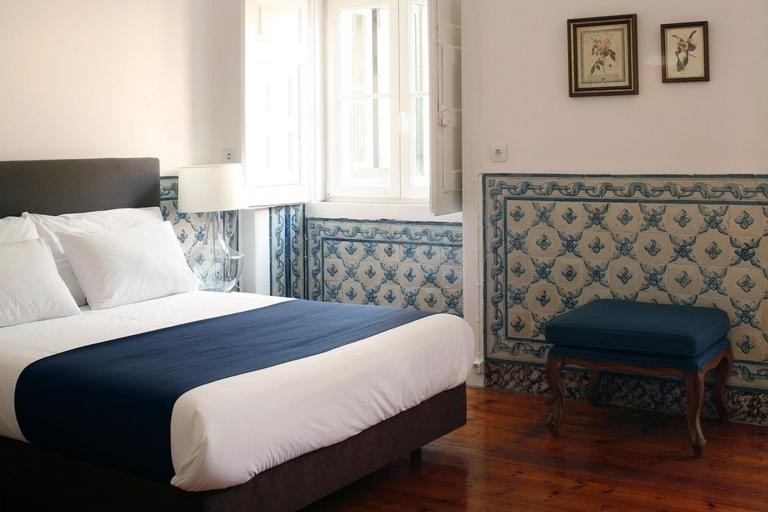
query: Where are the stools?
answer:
[542,298,735,454]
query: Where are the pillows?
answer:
[0,205,201,325]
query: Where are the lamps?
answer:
[174,164,246,292]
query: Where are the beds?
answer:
[0,158,477,512]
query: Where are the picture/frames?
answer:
[567,14,638,98]
[661,20,711,85]
